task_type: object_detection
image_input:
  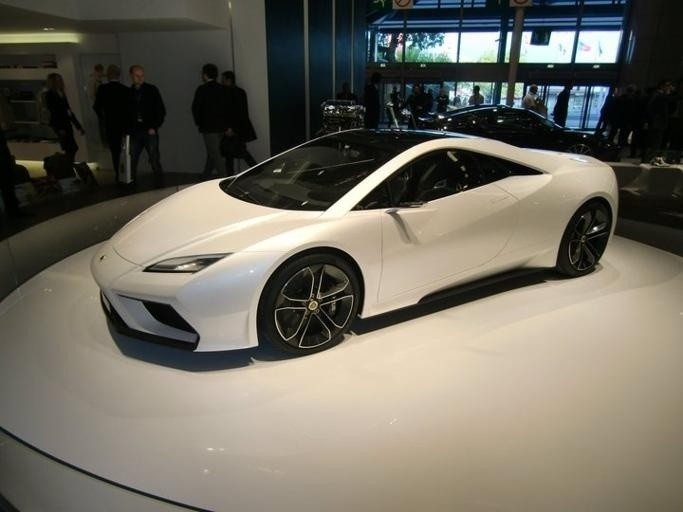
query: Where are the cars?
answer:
[431,101,457,113]
[319,98,415,160]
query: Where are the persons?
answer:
[389,83,450,130]
[453,94,467,106]
[468,85,484,105]
[337,74,383,127]
[549,78,573,131]
[521,84,538,113]
[1,102,36,222]
[37,62,257,186]
[598,80,683,170]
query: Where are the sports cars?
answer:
[419,105,628,163]
[84,124,622,365]
